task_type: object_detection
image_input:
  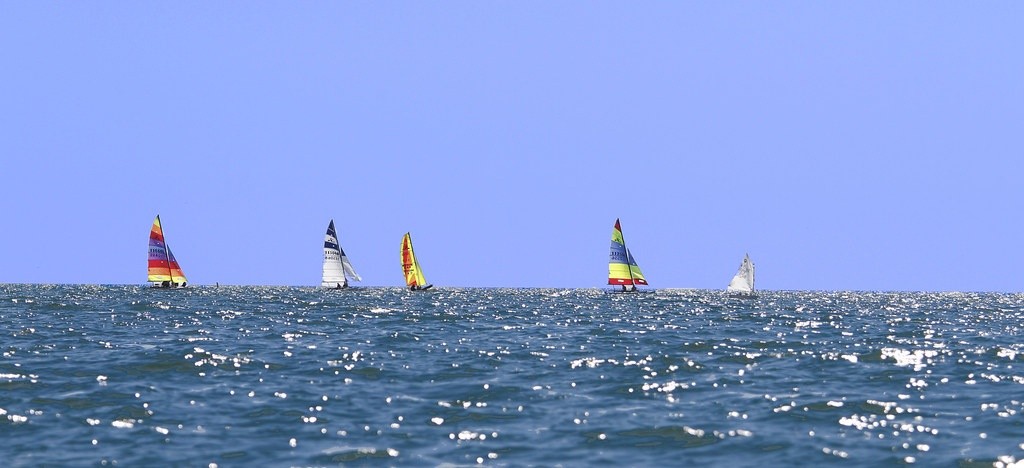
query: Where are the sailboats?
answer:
[399,232,433,291]
[319,220,362,290]
[604,218,656,297]
[727,253,759,293]
[145,214,190,291]
